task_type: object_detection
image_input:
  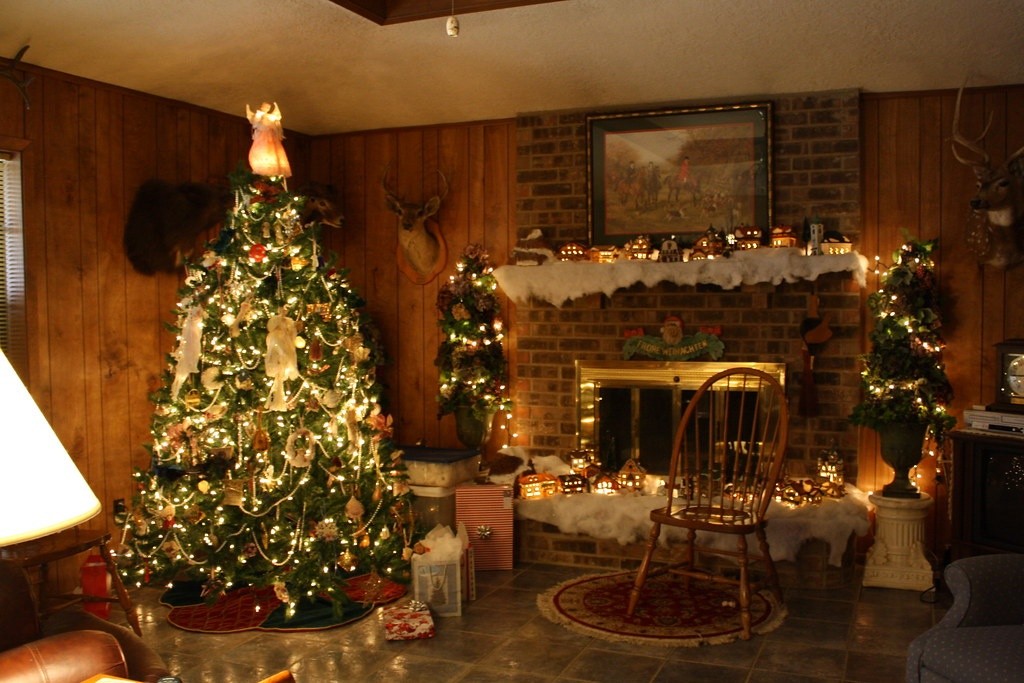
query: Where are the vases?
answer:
[879,420,930,494]
[452,404,496,469]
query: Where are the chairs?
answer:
[628,368,790,641]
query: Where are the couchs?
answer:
[904,554,1024,683]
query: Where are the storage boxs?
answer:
[381,446,514,640]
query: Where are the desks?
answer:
[942,425,1024,598]
[0,528,143,637]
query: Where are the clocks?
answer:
[992,340,1024,409]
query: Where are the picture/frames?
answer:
[585,101,776,246]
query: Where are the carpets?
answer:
[536,564,789,647]
